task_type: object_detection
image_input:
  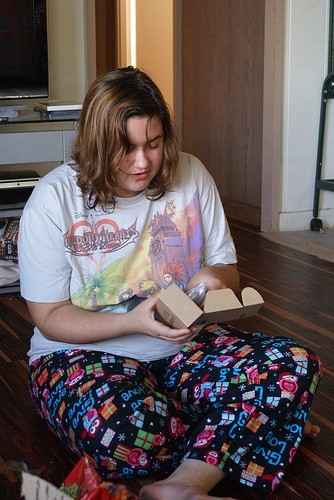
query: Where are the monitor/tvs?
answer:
[0,0,50,100]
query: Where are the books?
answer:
[36,100,85,112]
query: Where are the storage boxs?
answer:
[0,170,42,205]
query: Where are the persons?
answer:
[17,66,324,500]
